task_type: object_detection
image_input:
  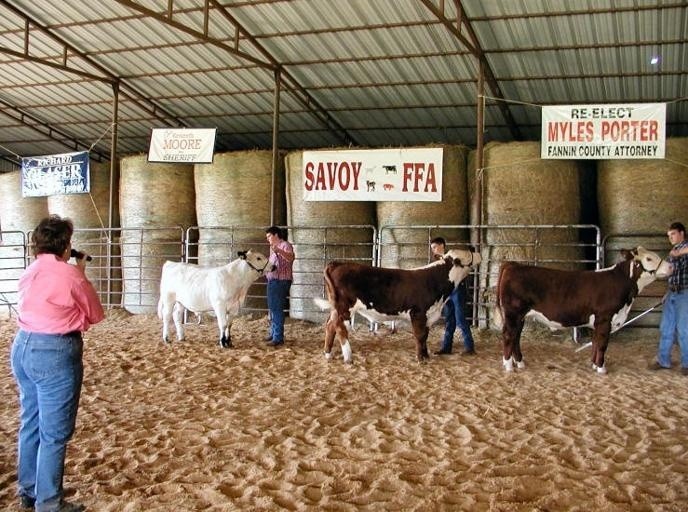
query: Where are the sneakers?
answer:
[459,349,475,355]
[263,335,284,346]
[434,350,450,354]
[647,363,688,375]
[20,487,85,512]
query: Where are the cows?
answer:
[493,245,675,374]
[157,247,277,349]
[322,248,481,365]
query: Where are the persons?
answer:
[261,226,294,346]
[429,236,476,356]
[10,215,105,512]
[651,222,688,377]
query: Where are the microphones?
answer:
[70,249,92,261]
